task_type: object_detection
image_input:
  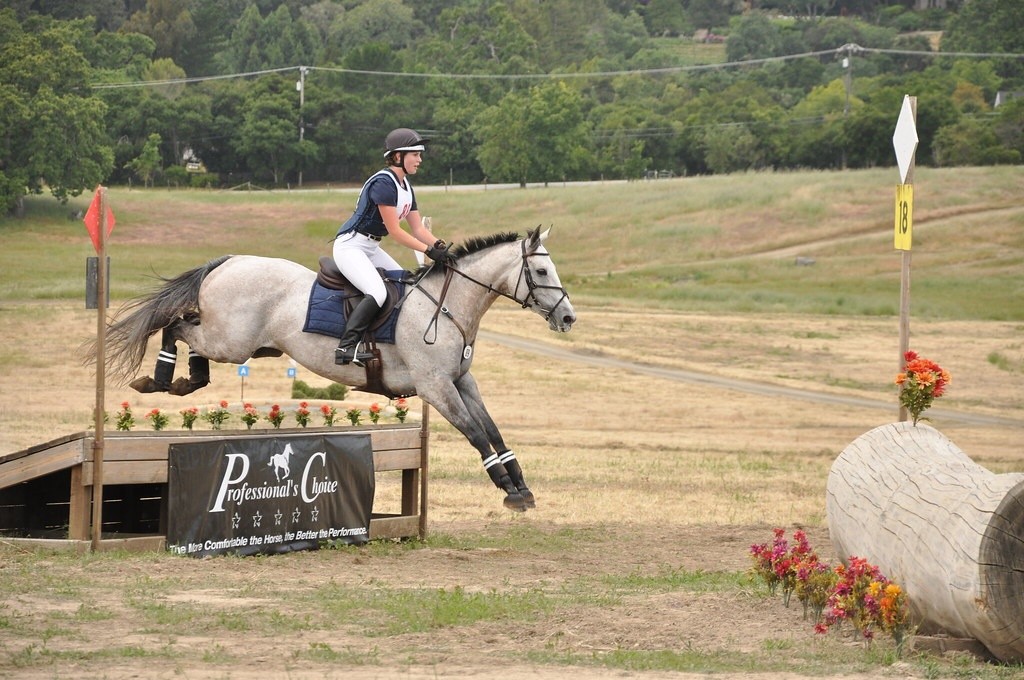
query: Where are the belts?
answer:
[354,228,381,241]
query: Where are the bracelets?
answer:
[434,238,446,248]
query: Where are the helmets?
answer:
[384,128,430,158]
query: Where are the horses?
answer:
[81,223,576,512]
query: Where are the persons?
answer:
[331,128,459,365]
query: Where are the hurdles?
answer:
[0,181,430,561]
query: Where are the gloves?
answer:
[434,239,456,266]
[424,245,446,262]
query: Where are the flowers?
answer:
[898,347,953,425]
[750,526,907,660]
[119,400,410,433]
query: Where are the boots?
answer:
[335,294,381,364]
